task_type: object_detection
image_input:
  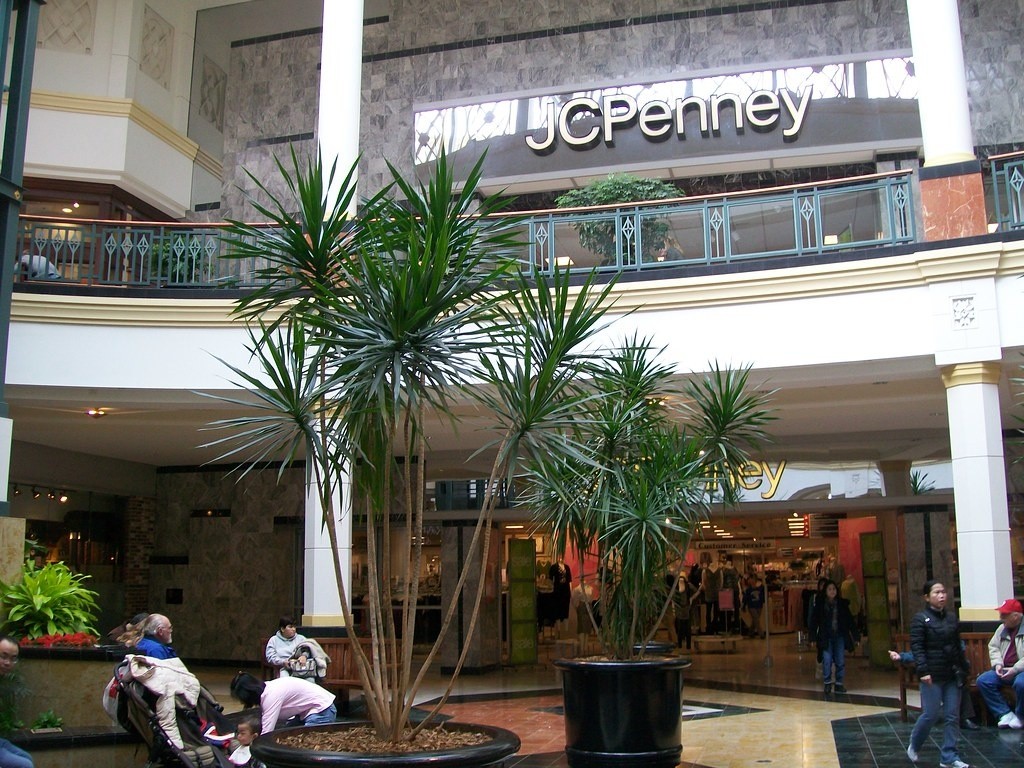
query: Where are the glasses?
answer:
[0,653,20,663]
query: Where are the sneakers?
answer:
[997,711,1015,729]
[940,760,969,768]
[1008,715,1023,730]
[907,744,920,763]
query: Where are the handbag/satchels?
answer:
[102,659,127,721]
[290,658,317,677]
[951,664,969,687]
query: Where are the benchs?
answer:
[260,634,402,714]
[895,632,1016,727]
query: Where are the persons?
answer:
[106,611,224,712]
[0,636,34,768]
[702,559,743,634]
[888,638,978,730]
[549,559,572,632]
[742,576,765,638]
[827,557,860,631]
[975,599,1024,728]
[265,615,318,683]
[573,580,593,651]
[228,712,266,768]
[672,571,697,649]
[907,578,970,768]
[230,672,337,735]
[808,577,860,693]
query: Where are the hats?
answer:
[994,599,1023,614]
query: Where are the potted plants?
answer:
[217,136,781,768]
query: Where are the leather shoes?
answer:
[960,719,980,730]
[824,684,831,695]
[834,684,847,692]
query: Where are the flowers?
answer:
[0,540,102,648]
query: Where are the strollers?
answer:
[114,660,270,768]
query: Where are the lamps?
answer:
[84,407,108,419]
[12,483,68,502]
